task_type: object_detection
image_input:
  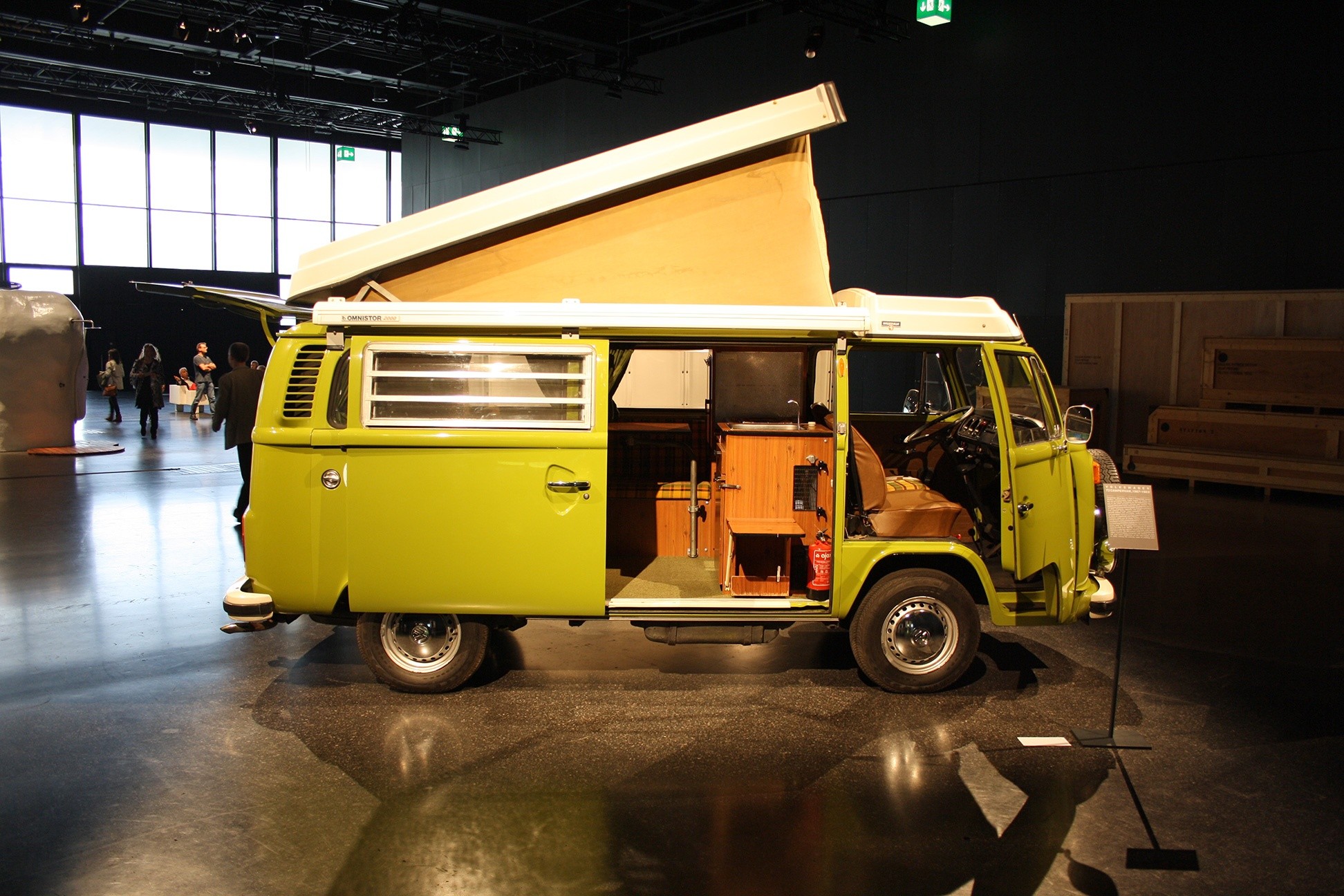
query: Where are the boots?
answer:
[113,414,122,422]
[105,414,114,421]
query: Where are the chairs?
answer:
[169,385,209,413]
[810,401,980,543]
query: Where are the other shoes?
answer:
[141,426,146,436]
[151,432,157,439]
[233,508,244,522]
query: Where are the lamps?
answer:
[63,0,92,25]
[301,1,326,12]
[244,120,257,135]
[605,86,623,101]
[454,141,470,151]
[801,24,824,60]
[176,12,281,41]
[313,127,332,135]
[192,60,215,76]
[372,87,388,104]
[855,35,876,46]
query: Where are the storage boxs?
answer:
[975,384,1110,453]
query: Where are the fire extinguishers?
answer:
[807,528,832,602]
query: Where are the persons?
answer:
[179,367,197,390]
[129,343,165,440]
[99,348,125,423]
[212,342,265,523]
[190,342,218,420]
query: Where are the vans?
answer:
[124,276,1126,697]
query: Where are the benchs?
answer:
[607,420,710,500]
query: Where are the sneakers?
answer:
[189,414,198,420]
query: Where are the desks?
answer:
[607,421,701,558]
[725,518,805,597]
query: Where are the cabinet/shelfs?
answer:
[713,433,834,594]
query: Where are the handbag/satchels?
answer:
[102,383,117,397]
